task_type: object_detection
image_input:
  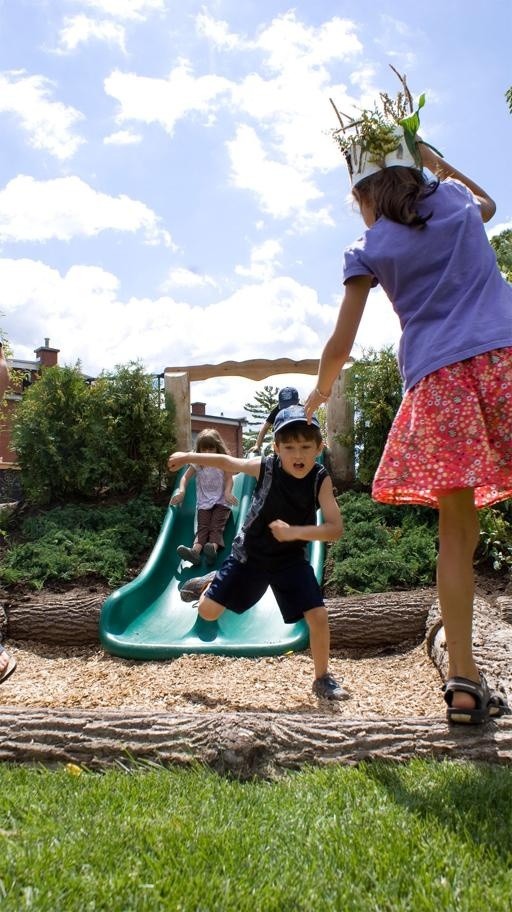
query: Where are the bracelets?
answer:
[315,384,334,400]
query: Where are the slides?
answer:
[99,450,324,659]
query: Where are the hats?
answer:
[278,387,299,410]
[271,404,321,433]
[345,125,422,190]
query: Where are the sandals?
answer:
[442,670,506,725]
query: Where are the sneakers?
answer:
[203,542,218,566]
[176,544,202,566]
[311,672,350,701]
[179,570,218,609]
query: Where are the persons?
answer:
[166,406,353,702]
[246,387,300,455]
[170,428,240,567]
[301,134,512,724]
[0,644,18,686]
